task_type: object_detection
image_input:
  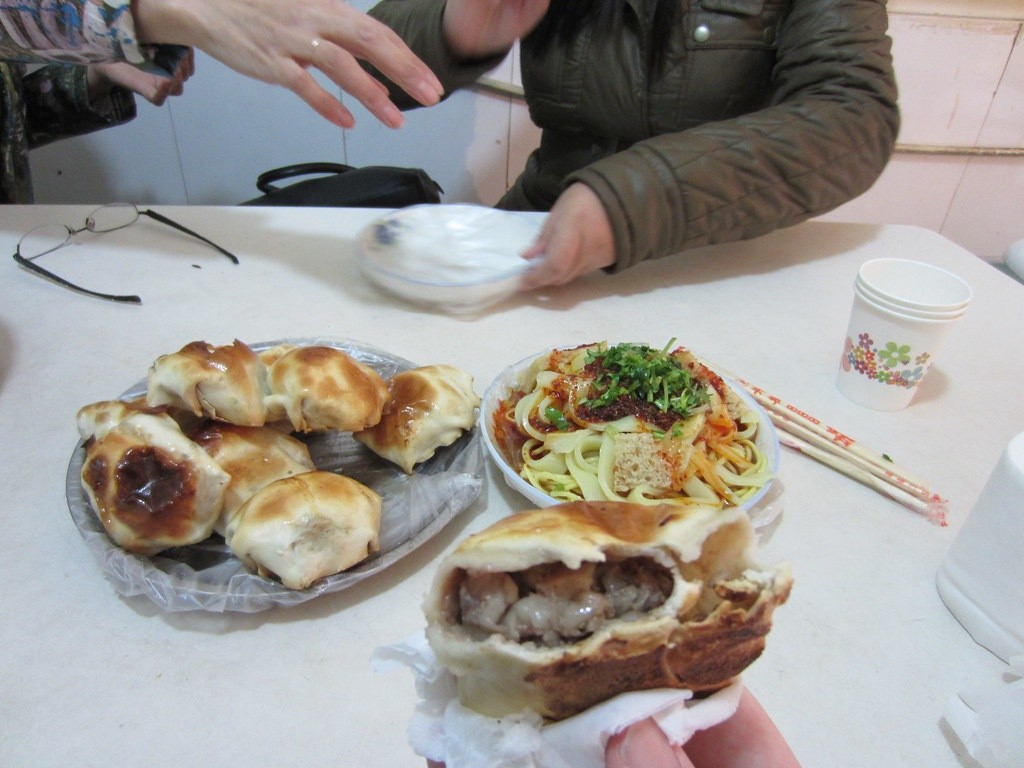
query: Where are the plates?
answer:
[64,336,485,610]
[353,204,544,312]
[479,342,782,520]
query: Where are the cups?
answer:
[838,258,970,412]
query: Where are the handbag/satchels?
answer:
[238,162,445,208]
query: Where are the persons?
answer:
[356,0,901,290]
[0,0,448,205]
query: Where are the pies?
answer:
[423,498,797,721]
[72,336,476,591]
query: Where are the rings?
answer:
[307,37,326,67]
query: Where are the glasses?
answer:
[13,202,239,304]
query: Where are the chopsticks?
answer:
[710,361,941,520]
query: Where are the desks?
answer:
[0,203,1024,768]
